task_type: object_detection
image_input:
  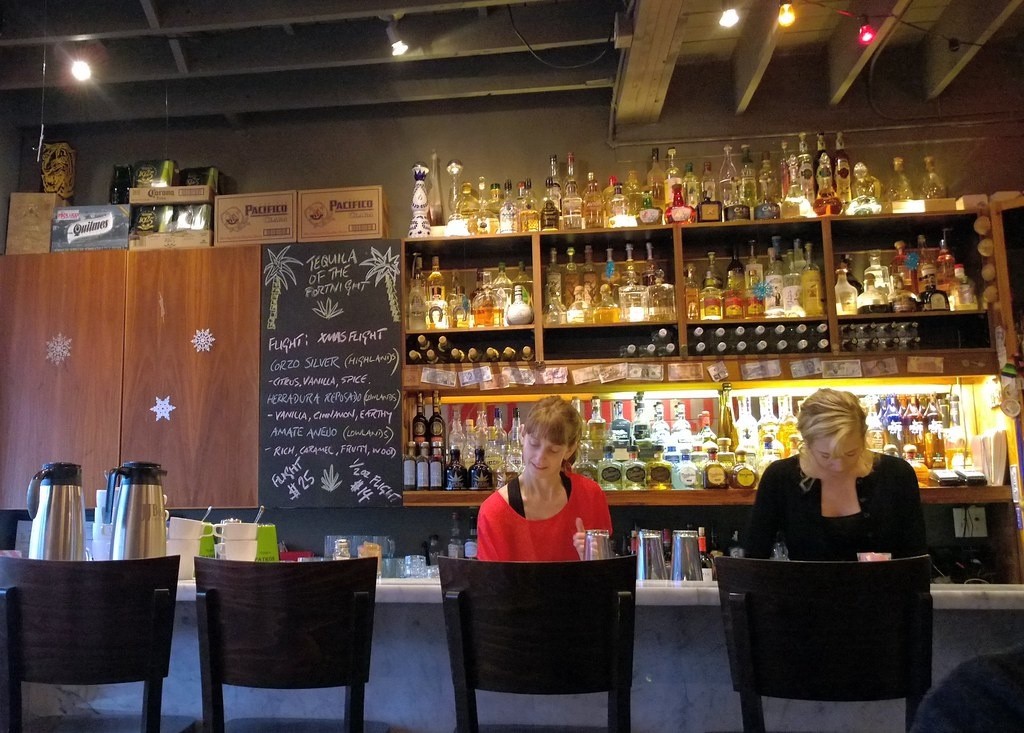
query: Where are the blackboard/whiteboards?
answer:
[254,236,404,509]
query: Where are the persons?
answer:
[744,388,925,561]
[476,395,612,560]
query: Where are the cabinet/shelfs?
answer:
[400,203,1023,506]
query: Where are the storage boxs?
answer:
[5,185,389,255]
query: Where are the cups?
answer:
[582,528,717,582]
[94,488,258,580]
[297,535,440,580]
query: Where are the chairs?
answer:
[716,554,934,733]
[194,556,378,733]
[436,555,639,733]
[0,555,181,733]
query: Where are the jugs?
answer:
[27,462,85,561]
[102,460,166,560]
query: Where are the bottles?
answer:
[405,232,979,363]
[408,131,949,239]
[402,384,979,493]
[421,505,790,567]
[109,163,134,205]
